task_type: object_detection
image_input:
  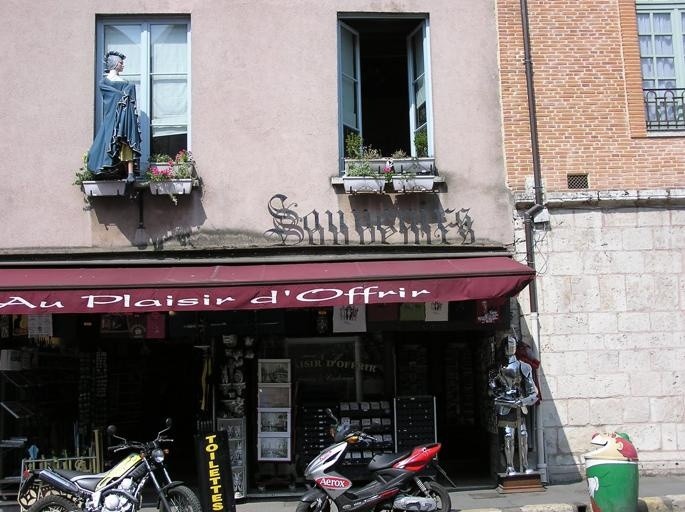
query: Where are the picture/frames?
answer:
[0,400,34,419]
[256,358,293,463]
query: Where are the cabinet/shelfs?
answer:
[0,368,37,501]
[216,416,248,504]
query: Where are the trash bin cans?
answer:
[583,432,639,512]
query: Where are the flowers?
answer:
[144,149,193,207]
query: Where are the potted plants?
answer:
[341,127,436,193]
[71,166,128,211]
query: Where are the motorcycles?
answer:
[20,417,202,512]
[295,407,456,512]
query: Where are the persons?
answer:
[98,49,138,161]
[485,334,542,474]
